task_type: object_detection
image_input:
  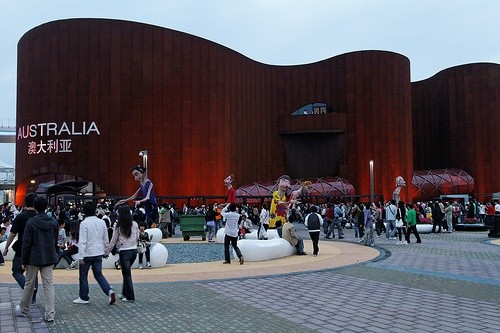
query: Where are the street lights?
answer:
[139,150,148,178]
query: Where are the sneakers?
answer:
[109,290,116,304]
[73,297,89,303]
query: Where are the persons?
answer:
[115,165,158,229]
[393,176,406,202]
[222,173,236,213]
[0,192,500,322]
[269,175,296,230]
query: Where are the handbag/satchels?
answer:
[259,226,268,238]
[137,239,146,253]
[395,219,403,227]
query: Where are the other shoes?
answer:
[121,298,134,302]
[324,232,398,247]
[146,262,151,267]
[45,317,55,321]
[415,241,420,243]
[403,241,407,244]
[298,252,307,254]
[70,261,78,267]
[16,305,26,316]
[223,261,230,264]
[240,256,244,264]
[139,265,143,269]
[396,241,402,244]
[118,294,124,298]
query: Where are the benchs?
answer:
[416,224,439,233]
[217,226,297,262]
[0,228,169,269]
